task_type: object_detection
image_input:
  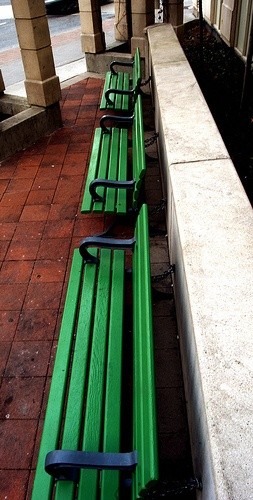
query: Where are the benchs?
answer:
[80,94,161,239]
[99,46,151,112]
[29,203,179,500]
